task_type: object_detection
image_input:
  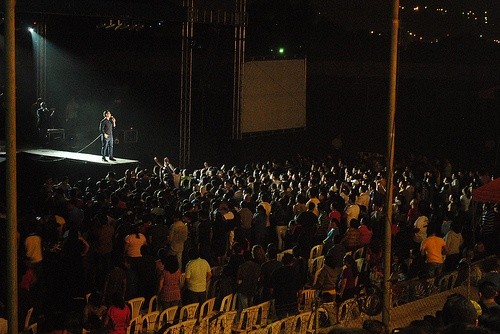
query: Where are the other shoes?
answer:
[110,159,116,161]
[102,158,107,161]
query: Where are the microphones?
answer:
[111,116,114,119]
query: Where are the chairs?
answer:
[127,245,358,334]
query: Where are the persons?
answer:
[99,110,116,161]
[31,98,81,141]
[0,152,500,334]
